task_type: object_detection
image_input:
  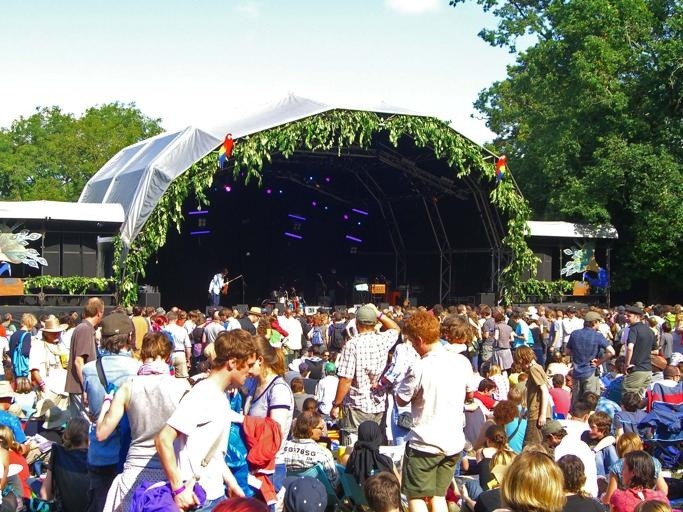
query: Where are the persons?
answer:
[207,267,227,307]
[1,294,683,512]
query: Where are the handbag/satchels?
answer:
[12,331,32,378]
[397,411,415,432]
[131,478,207,512]
[193,342,209,358]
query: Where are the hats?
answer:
[42,406,71,430]
[626,306,644,316]
[540,421,564,442]
[651,356,669,371]
[663,365,683,376]
[246,306,263,317]
[355,305,378,326]
[32,394,63,418]
[39,318,69,333]
[0,381,18,399]
[282,475,328,512]
[101,312,132,336]
[584,311,604,323]
[298,363,336,374]
[669,351,683,366]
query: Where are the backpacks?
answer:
[329,324,346,350]
[311,325,326,346]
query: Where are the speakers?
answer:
[206,305,223,317]
[330,305,347,315]
[261,307,272,317]
[476,293,495,307]
[375,303,389,311]
[138,292,160,307]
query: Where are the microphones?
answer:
[226,278,228,282]
